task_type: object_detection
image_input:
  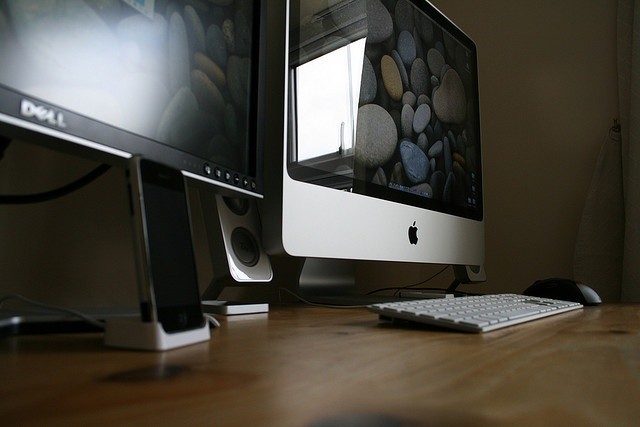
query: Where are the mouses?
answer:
[522,277,603,306]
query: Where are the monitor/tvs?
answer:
[280,0,487,303]
[0,0,269,338]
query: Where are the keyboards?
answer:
[365,293,584,334]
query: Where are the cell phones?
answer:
[128,155,205,334]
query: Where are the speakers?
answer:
[198,188,274,315]
[444,265,488,298]
